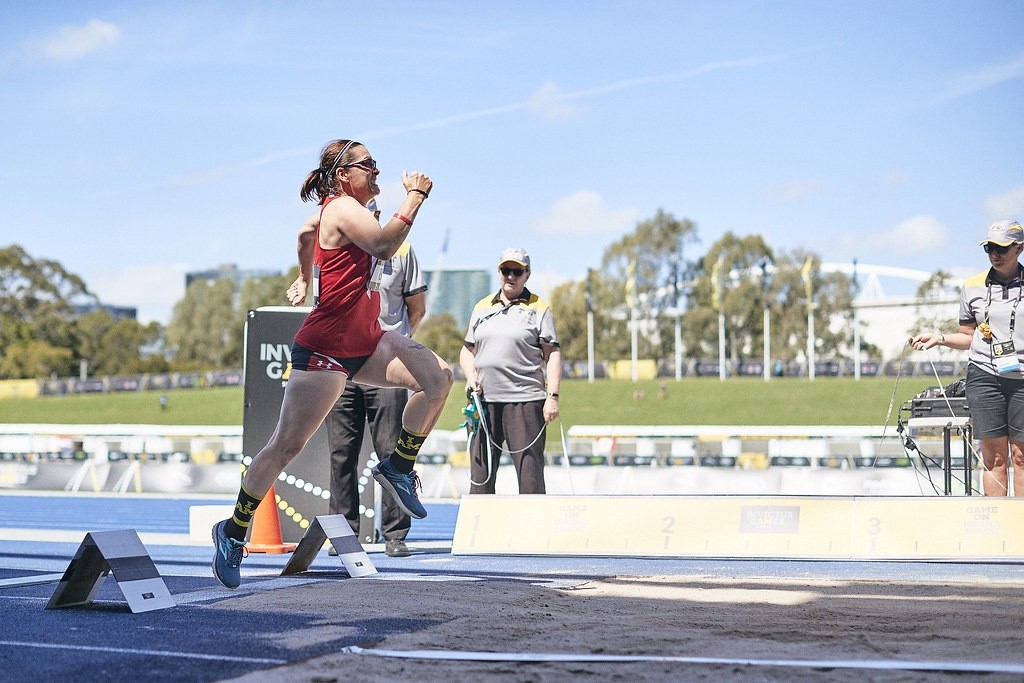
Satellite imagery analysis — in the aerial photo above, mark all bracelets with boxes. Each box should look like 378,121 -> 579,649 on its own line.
547,392 -> 559,397
407,189 -> 428,199
939,333 -> 945,345
393,213 -> 413,227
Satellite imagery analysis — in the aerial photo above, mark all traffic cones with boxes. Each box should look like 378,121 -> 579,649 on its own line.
244,483 -> 295,552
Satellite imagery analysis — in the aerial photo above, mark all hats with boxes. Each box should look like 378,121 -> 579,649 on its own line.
978,218 -> 1024,247
365,196 -> 380,216
496,247 -> 530,269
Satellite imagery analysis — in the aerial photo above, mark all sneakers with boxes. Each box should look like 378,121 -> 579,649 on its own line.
212,518 -> 248,588
372,457 -> 428,520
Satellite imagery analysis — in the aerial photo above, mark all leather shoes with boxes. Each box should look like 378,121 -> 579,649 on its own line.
327,546 -> 337,556
384,539 -> 410,557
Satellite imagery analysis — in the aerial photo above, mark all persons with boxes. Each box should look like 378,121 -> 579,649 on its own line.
908,220 -> 1024,496
459,247 -> 562,494
324,198 -> 429,558
213,137 -> 455,589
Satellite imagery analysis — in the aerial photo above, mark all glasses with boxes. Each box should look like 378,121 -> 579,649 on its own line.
984,244 -> 1015,253
346,159 -> 377,169
501,268 -> 527,276
